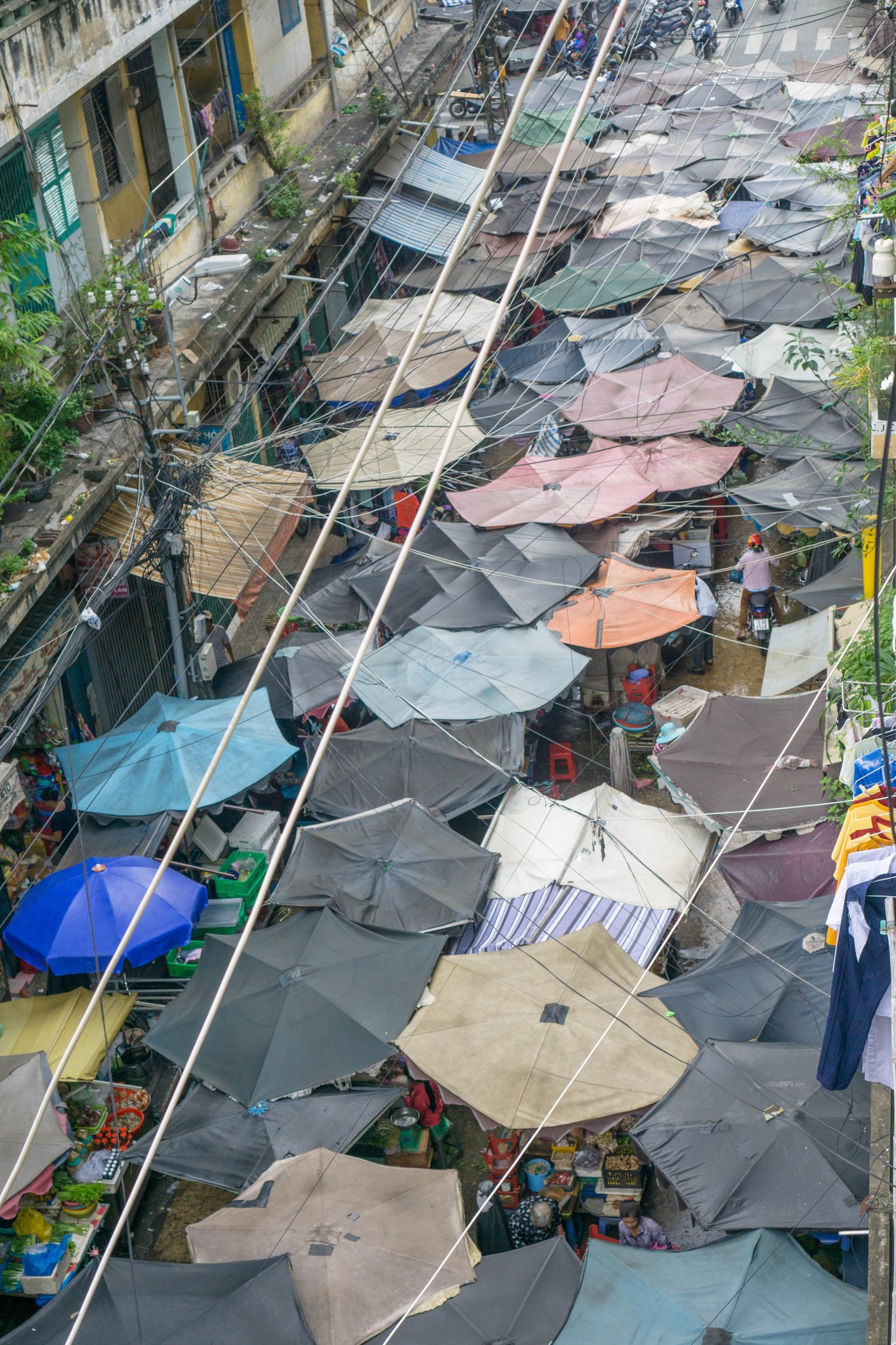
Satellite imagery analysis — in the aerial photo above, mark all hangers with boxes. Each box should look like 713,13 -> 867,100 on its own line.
400,482 -> 413,494
850,694 -> 896,910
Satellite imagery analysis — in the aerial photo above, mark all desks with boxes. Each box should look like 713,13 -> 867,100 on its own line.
385,1129 -> 433,1171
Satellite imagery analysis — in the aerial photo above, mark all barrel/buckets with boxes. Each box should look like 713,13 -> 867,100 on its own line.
525,1158 -> 551,1192
120,1046 -> 154,1085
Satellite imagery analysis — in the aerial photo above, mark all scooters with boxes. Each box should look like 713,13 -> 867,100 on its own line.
723,0 -> 741,28
768,0 -> 784,13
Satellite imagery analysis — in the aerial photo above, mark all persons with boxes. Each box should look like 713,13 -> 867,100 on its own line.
30,787 -> 78,855
346,511 -> 378,547
458,131 -> 469,142
611,0 -> 626,46
618,1197 -> 673,1251
734,533 -> 785,640
530,306 -> 553,338
572,23 -> 599,71
736,0 -> 746,21
687,577 -> 719,675
689,0 -> 713,49
310,708 -> 351,735
203,609 -> 235,668
603,57 -> 621,82
506,1193 -> 559,1249
554,16 -> 571,69
248,770 -> 302,824
401,1062 -> 451,1140
465,125 -> 478,142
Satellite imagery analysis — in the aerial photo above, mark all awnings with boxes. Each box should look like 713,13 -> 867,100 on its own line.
86,434 -> 314,626
347,182 -> 483,265
372,131 -> 494,206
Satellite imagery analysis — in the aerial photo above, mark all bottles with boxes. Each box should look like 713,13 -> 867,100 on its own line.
103,1149 -> 121,1180
199,872 -> 218,900
94,1127 -> 133,1153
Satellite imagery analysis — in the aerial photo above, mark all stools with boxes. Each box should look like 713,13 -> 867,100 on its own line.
480,1132 -> 527,1209
429,1123 -> 464,1169
562,1212 -> 623,1262
620,660 -> 658,711
547,742 -> 578,786
713,512 -> 730,545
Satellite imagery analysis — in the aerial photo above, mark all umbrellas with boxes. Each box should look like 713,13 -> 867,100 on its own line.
0,46 -> 884,1345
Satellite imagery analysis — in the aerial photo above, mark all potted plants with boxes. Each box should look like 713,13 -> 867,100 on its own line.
0,210 -> 175,526
223,83 -> 332,224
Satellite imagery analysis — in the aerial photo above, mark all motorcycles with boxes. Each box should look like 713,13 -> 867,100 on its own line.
690,12 -> 718,61
449,0 -> 696,121
734,556 -> 782,657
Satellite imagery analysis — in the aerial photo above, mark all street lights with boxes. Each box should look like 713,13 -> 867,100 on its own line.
164,252 -> 249,442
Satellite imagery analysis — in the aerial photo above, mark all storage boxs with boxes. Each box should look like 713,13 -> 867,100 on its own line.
543,1131 -> 647,1193
83,1148 -> 131,1195
650,684 -> 710,735
163,809 -> 282,981
18,1242 -> 72,1296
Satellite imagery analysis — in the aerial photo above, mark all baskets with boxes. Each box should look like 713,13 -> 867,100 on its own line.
87,1111 -> 108,1137
349,1120 -> 386,1157
166,941 -> 204,978
0,1209 -> 24,1236
74,1106 -> 107,1133
214,850 -> 268,908
602,1154 -> 645,1189
61,1193 -> 97,1219
104,1108 -> 144,1134
192,899 -> 247,938
